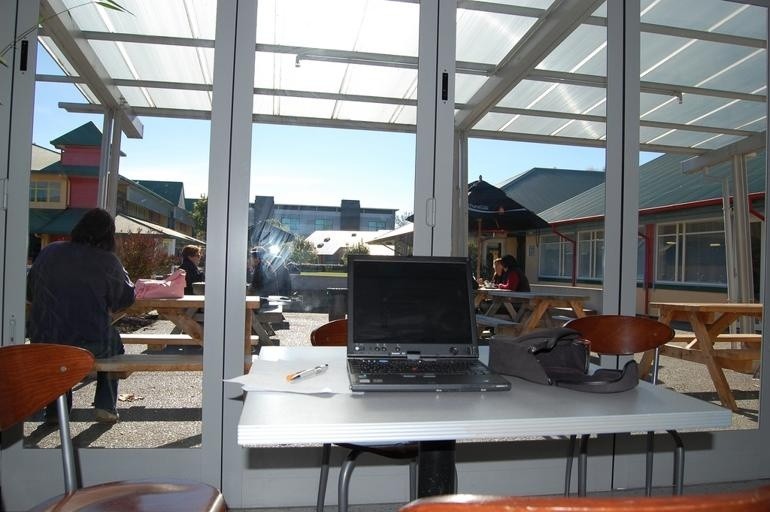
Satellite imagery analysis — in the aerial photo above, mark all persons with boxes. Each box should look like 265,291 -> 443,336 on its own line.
479,255 -> 531,336
176,245 -> 205,314
472,273 -> 479,290
26,207 -> 134,423
479,258 -> 508,288
271,256 -> 292,295
247,245 -> 279,314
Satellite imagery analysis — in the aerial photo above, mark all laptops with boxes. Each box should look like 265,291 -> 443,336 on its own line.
346,255 -> 512,391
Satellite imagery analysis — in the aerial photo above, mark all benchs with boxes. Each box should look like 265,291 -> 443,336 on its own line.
98,333 -> 201,371
476,308 -> 762,376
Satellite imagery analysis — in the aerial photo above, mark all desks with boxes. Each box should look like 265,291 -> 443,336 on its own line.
475,291 -> 591,340
635,301 -> 763,409
236,344 -> 734,512
120,294 -> 261,374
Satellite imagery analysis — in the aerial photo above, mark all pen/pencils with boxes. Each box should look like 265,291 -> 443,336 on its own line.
289,363 -> 328,381
286,369 -> 307,380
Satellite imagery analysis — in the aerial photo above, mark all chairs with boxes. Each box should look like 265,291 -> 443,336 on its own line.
1,344 -> 230,512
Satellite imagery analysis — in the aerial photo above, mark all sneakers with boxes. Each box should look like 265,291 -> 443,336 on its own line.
94,408 -> 120,424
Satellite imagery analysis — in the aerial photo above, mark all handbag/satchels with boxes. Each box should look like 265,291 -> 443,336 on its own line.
487,328 -> 639,394
133,268 -> 187,300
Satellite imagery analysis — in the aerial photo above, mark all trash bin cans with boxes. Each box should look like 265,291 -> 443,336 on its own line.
326,288 -> 348,322
